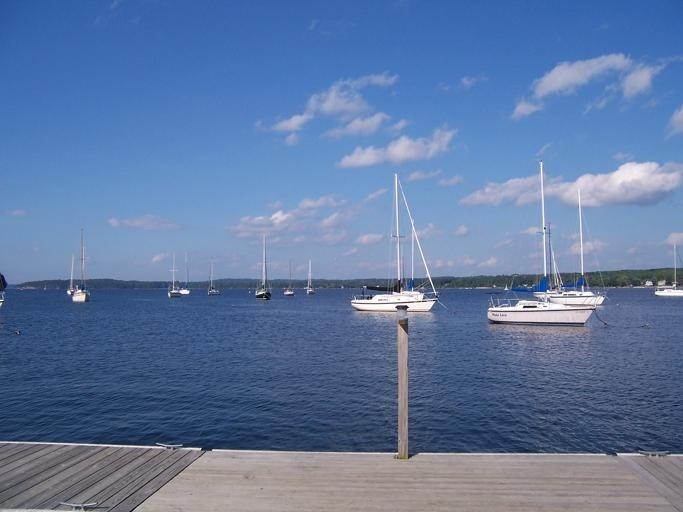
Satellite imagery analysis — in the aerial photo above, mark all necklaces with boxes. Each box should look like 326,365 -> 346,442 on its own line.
487,161 -> 607,324
66,233 -> 90,303
349,173 -> 439,312
303,259 -> 316,295
654,245 -> 682,297
167,251 -> 190,298
283,261 -> 293,296
254,235 -> 271,300
207,264 -> 220,296
